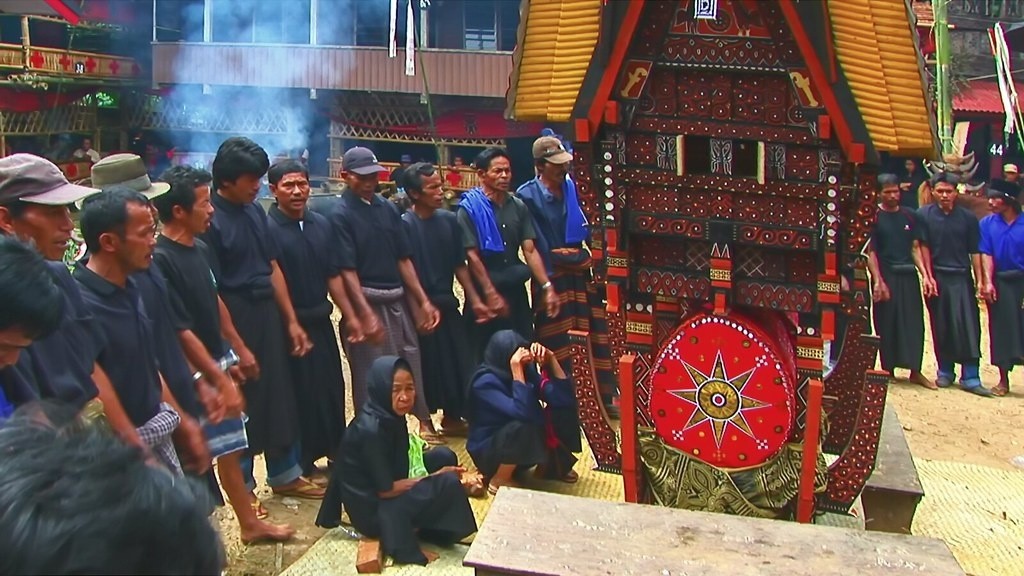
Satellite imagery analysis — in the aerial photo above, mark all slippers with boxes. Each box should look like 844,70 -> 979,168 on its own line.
278,484 -> 326,498
488,487 -> 498,495
420,431 -> 446,444
991,386 -> 1009,396
534,465 -> 579,483
438,423 -> 470,436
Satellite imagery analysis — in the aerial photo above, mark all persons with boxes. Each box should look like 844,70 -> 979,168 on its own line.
1001,165 -> 1024,205
915,170 -> 989,396
865,172 -> 938,390
0,138 -> 614,575
978,176 -> 1024,397
900,157 -> 924,210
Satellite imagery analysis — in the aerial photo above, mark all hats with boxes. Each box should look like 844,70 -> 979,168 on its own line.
342,146 -> 389,176
533,137 -> 573,164
0,152 -> 102,207
1004,164 -> 1018,172
75,152 -> 171,209
401,154 -> 412,162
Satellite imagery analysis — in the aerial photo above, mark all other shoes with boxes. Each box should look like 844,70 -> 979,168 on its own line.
936,375 -> 953,388
970,385 -> 991,396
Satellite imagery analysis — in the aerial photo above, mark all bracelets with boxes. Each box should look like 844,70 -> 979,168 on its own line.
542,280 -> 552,289
191,370 -> 204,383
